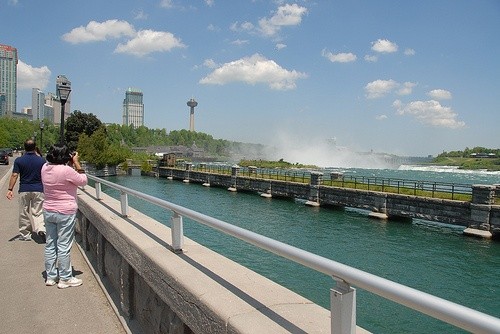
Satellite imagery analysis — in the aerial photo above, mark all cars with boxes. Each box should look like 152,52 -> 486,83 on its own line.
0,150 -> 9,165
4,148 -> 13,156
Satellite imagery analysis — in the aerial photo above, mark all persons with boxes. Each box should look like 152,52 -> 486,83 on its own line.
6,138 -> 47,242
34,145 -> 42,157
38,142 -> 88,289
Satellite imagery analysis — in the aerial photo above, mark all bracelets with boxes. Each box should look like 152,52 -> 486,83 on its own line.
8,189 -> 12,191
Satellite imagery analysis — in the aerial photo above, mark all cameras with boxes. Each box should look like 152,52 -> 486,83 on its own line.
68,153 -> 75,158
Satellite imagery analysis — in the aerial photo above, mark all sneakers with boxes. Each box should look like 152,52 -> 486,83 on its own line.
57,277 -> 83,289
45,278 -> 59,286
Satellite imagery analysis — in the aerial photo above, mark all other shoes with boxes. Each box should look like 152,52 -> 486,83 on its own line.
38,231 -> 46,243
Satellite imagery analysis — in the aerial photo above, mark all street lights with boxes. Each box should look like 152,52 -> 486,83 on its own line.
58,81 -> 72,144
39,120 -> 45,157
